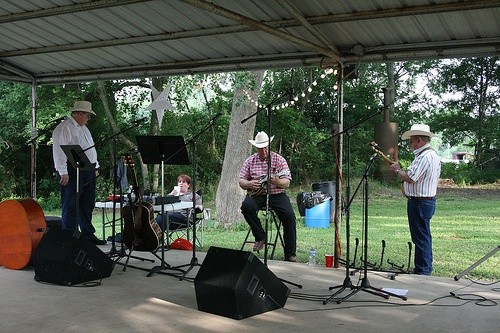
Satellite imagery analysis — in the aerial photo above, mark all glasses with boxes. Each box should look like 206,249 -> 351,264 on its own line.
87,114 -> 91,120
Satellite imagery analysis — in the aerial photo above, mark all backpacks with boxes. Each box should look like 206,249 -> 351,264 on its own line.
170,238 -> 192,251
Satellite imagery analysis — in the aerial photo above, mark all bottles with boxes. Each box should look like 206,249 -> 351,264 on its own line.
309,248 -> 316,266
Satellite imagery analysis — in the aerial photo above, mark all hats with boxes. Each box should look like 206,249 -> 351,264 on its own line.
70,100 -> 96,116
400,123 -> 438,140
247,130 -> 274,149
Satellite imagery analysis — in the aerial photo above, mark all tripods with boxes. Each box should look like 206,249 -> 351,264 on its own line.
83,120 -> 216,281
323,111 -> 409,306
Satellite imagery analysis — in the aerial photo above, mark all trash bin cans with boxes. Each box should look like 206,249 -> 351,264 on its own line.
312,181 -> 336,223
303,191 -> 332,230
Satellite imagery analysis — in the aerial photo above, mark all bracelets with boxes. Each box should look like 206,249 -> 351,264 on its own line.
395,169 -> 401,174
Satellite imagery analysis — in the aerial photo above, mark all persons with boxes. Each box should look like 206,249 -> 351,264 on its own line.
52,101 -> 108,245
154,175 -> 202,233
388,123 -> 442,275
238,130 -> 297,262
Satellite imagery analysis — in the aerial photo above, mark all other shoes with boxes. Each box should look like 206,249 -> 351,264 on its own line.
253,238 -> 266,251
285,256 -> 297,262
80,233 -> 107,245
70,227 -> 80,239
107,235 -> 120,242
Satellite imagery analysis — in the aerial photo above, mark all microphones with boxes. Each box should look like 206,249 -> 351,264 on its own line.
134,117 -> 149,123
209,113 -> 222,123
51,116 -> 68,122
284,88 -> 291,94
375,105 -> 391,111
370,153 -> 378,161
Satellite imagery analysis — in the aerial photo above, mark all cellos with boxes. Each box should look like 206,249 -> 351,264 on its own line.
0,198 -> 47,270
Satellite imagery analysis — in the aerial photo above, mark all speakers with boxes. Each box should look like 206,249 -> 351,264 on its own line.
194,246 -> 291,319
32,215 -> 116,286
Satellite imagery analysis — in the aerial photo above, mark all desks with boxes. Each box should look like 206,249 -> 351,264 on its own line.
95,201 -> 195,251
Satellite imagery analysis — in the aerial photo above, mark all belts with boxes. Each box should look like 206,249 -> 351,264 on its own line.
409,196 -> 433,200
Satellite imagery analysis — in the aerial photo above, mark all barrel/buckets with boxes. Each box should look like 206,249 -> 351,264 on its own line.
311,181 -> 336,223
305,199 -> 329,228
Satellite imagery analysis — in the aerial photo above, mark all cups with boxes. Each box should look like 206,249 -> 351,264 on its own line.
325,255 -> 333,267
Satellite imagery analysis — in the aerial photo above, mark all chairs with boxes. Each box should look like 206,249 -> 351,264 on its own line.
163,189 -> 205,248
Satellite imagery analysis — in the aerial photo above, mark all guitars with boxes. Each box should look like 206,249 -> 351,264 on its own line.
121,152 -> 162,253
245,169 -> 291,197
370,141 -> 410,199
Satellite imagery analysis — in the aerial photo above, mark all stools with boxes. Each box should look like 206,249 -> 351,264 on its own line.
240,207 -> 284,260
